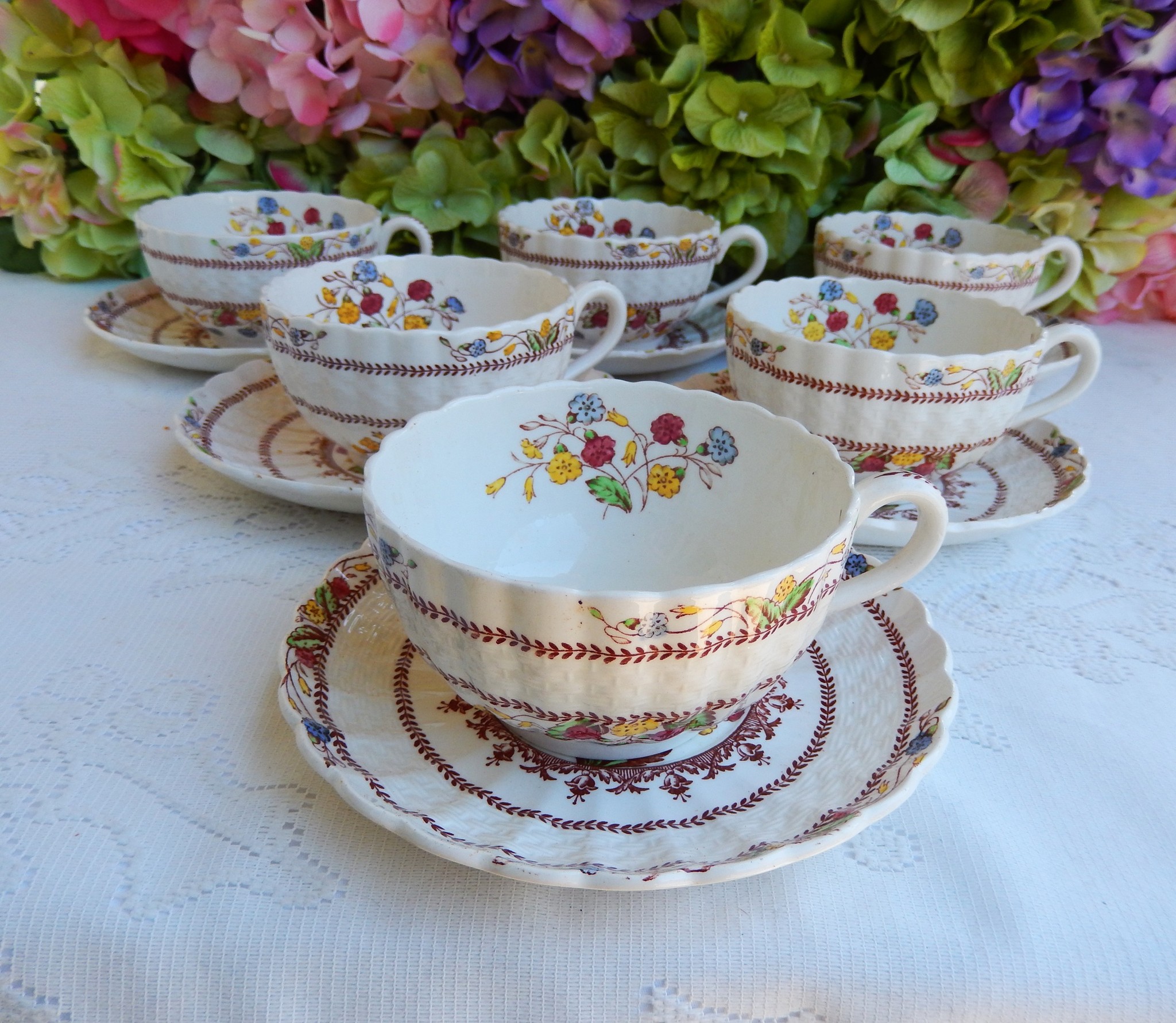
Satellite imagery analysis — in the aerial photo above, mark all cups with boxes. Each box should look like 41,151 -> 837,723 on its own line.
132,189 -> 434,348
814,209 -> 1085,319
497,198 -> 768,346
259,254 -> 626,468
360,380 -> 951,764
726,275 -> 1104,480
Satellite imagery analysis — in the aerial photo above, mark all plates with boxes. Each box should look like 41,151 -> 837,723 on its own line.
171,355 -> 613,512
83,275 -> 274,374
670,366 -> 1089,549
280,535 -> 960,892
1022,310 -> 1080,381
564,305 -> 729,375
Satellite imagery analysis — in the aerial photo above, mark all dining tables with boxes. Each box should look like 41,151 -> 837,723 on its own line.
0,258 -> 1175,1023
